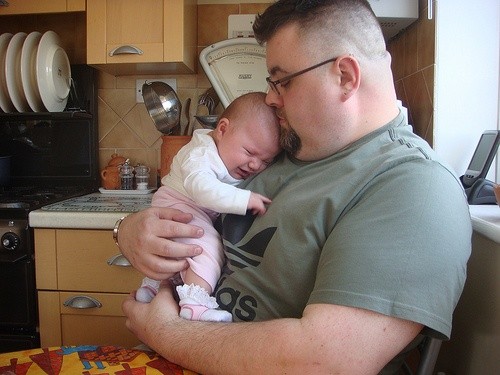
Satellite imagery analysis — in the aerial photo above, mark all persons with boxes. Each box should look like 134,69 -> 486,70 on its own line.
139,0 -> 473,375
140,91 -> 280,324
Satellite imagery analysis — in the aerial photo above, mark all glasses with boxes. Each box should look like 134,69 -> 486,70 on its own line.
265,57 -> 336,96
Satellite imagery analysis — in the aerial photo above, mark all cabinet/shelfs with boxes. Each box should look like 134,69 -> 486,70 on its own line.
35,228 -> 152,347
0,0 -> 196,74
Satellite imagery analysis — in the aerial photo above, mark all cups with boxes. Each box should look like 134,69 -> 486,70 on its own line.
162,136 -> 192,184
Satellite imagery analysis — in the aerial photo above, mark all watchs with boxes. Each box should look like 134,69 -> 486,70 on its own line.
112,215 -> 128,245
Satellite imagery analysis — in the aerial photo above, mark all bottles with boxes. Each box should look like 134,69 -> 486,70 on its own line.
118,161 -> 133,190
135,166 -> 151,189
101,154 -> 127,190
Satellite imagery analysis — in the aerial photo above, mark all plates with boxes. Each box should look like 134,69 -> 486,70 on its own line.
5,32 -> 30,113
0,32 -> 15,114
20,31 -> 47,112
36,30 -> 71,112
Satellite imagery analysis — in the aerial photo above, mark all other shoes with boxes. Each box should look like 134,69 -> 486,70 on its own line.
176,284 -> 234,324
135,276 -> 161,304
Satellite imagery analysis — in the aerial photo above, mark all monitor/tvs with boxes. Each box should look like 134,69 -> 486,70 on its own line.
461,130 -> 500,185
